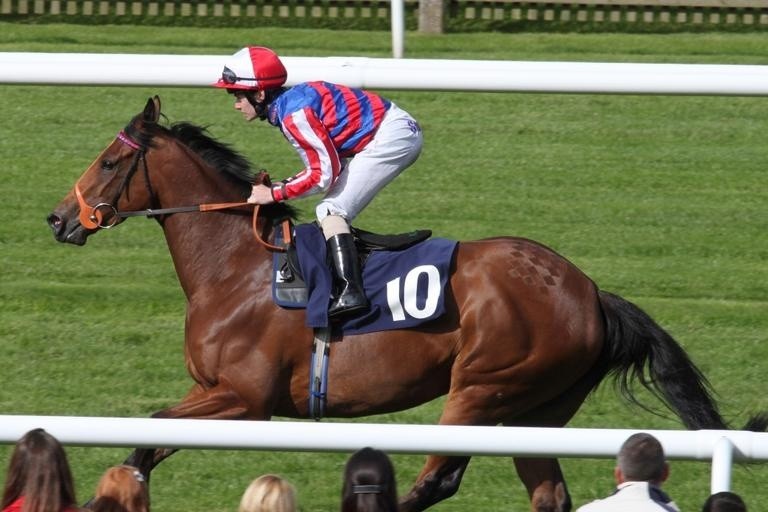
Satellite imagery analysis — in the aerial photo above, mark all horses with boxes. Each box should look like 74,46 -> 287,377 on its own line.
46,92 -> 768,512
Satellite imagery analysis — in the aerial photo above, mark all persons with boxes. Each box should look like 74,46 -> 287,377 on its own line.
0,427 -> 92,512
236,473 -> 298,512
338,448 -> 399,511
78,463 -> 152,512
210,45 -> 423,319
573,433 -> 682,512
702,491 -> 748,512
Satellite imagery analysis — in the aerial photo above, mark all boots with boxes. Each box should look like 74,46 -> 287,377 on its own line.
327,233 -> 371,319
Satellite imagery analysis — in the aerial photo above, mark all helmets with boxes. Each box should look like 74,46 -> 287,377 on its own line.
209,46 -> 287,90
